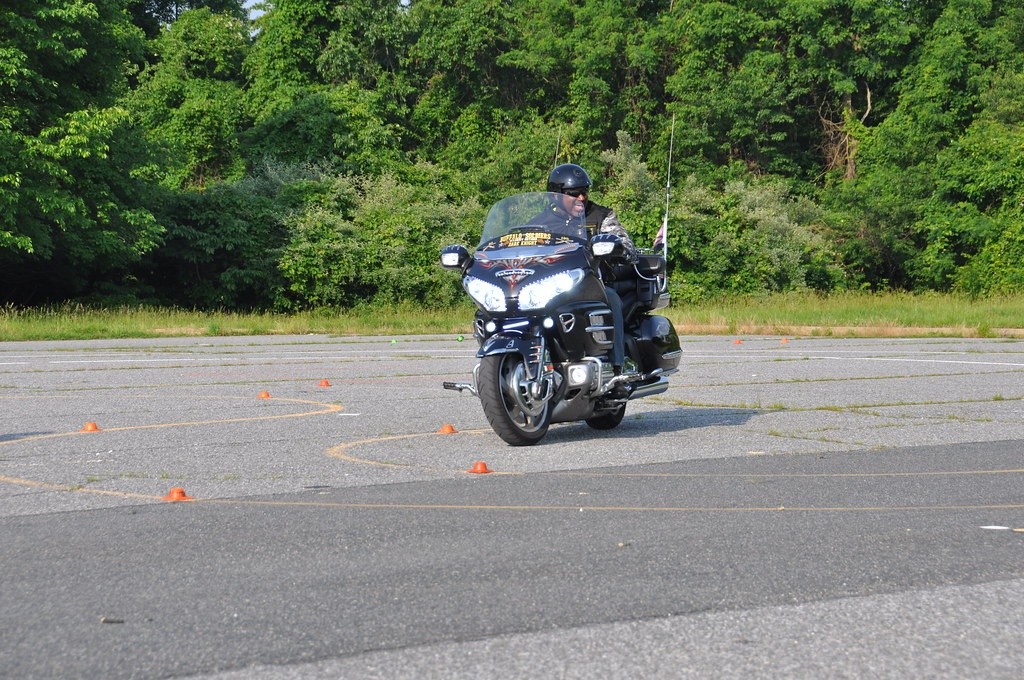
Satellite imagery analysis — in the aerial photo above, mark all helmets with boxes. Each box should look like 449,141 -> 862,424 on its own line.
547,164 -> 591,189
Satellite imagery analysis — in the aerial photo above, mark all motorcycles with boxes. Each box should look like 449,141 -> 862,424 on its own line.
440,191 -> 683,446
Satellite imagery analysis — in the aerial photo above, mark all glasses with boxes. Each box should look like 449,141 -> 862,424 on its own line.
562,190 -> 588,197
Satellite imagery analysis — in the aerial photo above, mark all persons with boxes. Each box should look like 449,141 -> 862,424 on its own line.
523,164 -> 637,402
